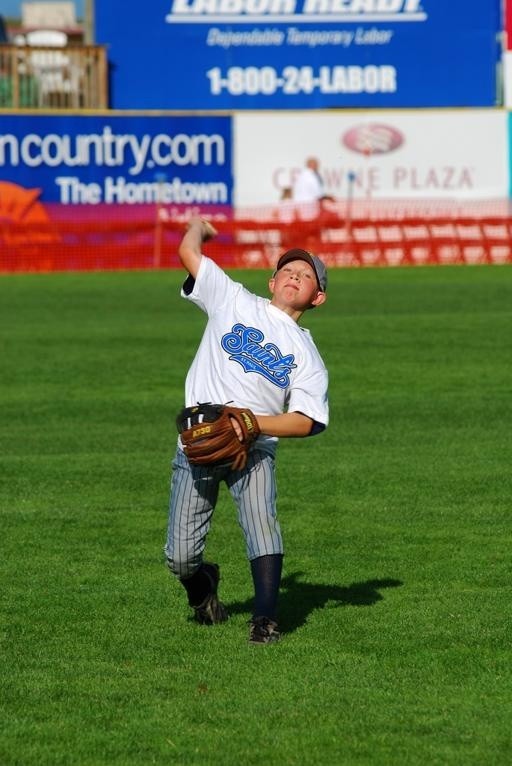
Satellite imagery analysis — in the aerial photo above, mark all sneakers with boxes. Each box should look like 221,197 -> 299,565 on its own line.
186,562 -> 223,624
247,615 -> 278,644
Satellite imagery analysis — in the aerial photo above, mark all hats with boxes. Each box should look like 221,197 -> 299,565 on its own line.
272,248 -> 327,292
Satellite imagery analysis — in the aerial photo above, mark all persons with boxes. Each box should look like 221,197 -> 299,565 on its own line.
168,214 -> 329,645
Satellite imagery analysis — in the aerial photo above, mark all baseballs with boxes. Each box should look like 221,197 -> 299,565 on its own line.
203,219 -> 217,240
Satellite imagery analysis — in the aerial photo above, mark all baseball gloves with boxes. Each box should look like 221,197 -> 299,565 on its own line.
180,404 -> 259,472
